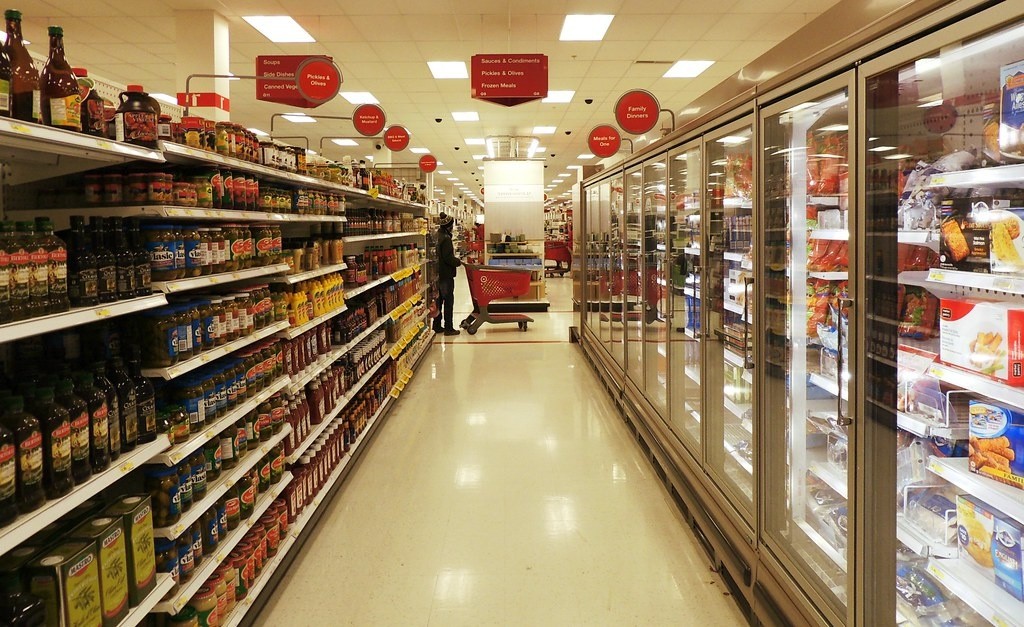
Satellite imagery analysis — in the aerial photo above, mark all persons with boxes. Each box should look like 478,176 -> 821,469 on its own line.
432,211 -> 462,336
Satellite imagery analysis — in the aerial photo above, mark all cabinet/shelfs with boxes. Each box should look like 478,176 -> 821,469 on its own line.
0,0 -> 440,627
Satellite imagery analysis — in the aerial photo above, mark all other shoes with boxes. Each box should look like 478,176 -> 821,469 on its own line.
444,329 -> 460,336
432,327 -> 444,333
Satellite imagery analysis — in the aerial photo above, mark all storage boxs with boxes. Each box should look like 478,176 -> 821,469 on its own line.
981,99 -> 1003,168
490,259 -> 498,265
36,517 -> 82,547
999,58 -> 1024,163
956,494 -> 1024,602
523,259 -> 532,264
0,543 -> 49,596
968,397 -> 1024,490
940,197 -> 1011,273
68,515 -> 129,627
25,537 -> 104,627
67,496 -> 106,517
102,492 -> 157,606
515,259 -> 522,265
506,259 -> 515,265
532,259 -> 542,265
498,259 -> 506,265
988,206 -> 1024,276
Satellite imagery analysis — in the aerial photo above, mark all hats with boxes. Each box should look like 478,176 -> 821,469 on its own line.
440,212 -> 454,229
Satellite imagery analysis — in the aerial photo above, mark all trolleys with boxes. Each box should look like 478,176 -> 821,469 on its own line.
459,260 -> 534,335
